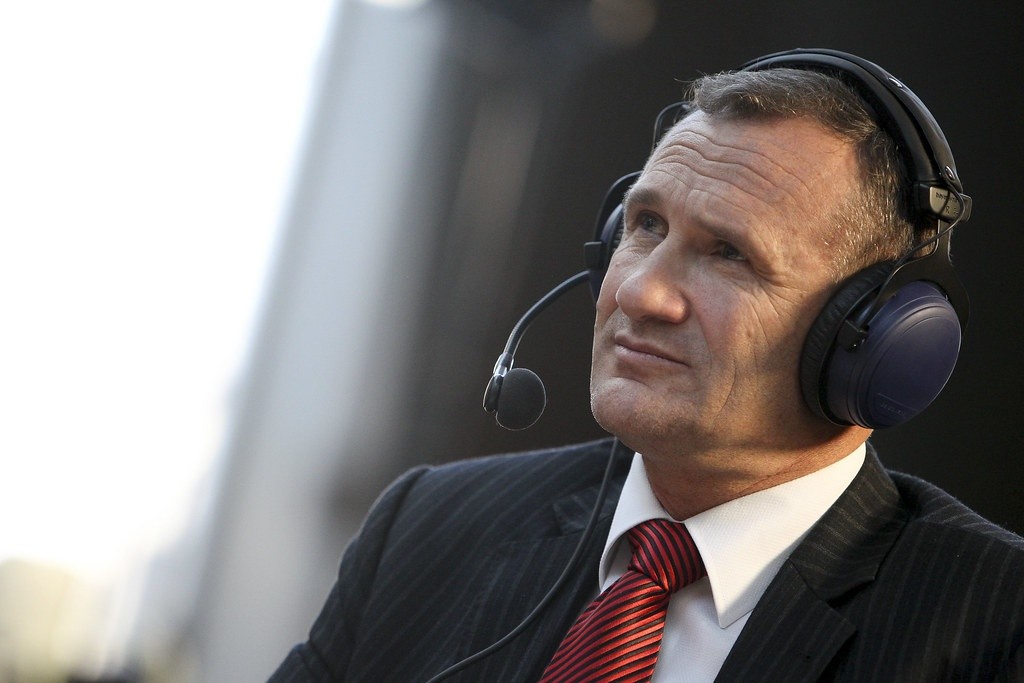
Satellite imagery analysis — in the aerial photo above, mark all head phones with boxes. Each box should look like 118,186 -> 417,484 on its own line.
579,47 -> 977,432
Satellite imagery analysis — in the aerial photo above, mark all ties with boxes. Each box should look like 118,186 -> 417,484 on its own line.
539,518 -> 708,683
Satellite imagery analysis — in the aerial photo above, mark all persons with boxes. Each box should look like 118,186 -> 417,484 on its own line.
266,63 -> 1024,683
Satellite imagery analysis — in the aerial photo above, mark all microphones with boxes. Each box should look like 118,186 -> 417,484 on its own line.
483,270 -> 587,433
